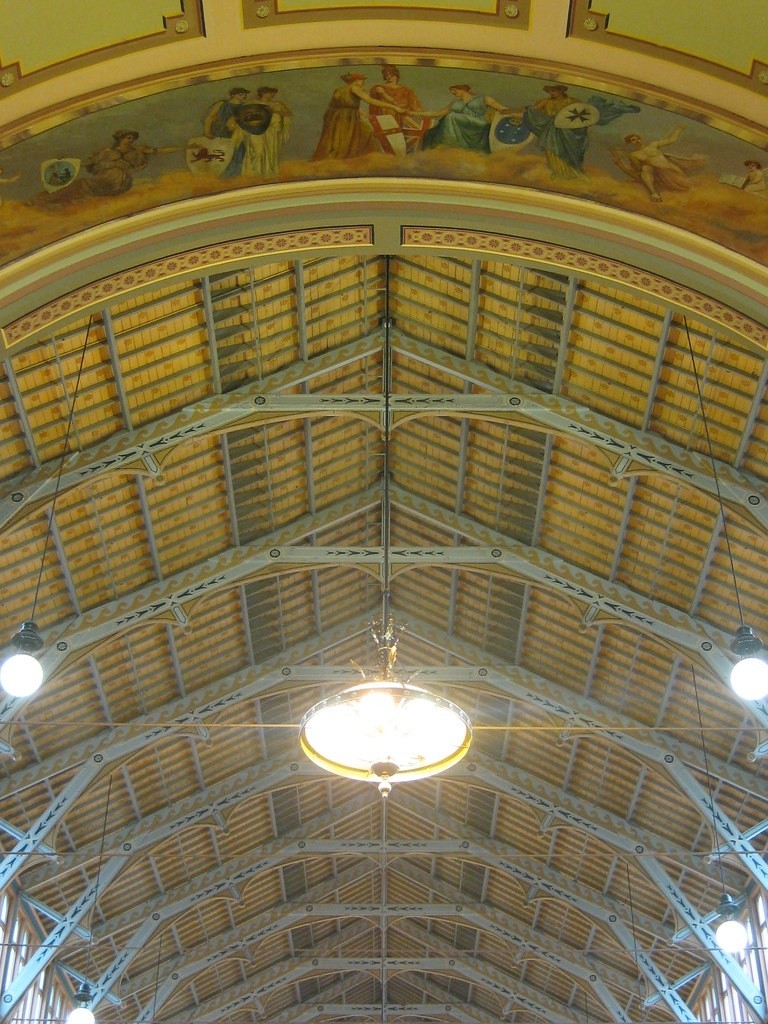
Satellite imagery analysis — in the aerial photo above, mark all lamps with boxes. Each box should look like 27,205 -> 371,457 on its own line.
61,774 -> 114,1024
296,254 -> 474,796
1,317 -> 91,697
682,310 -> 768,700
689,664 -> 750,953
